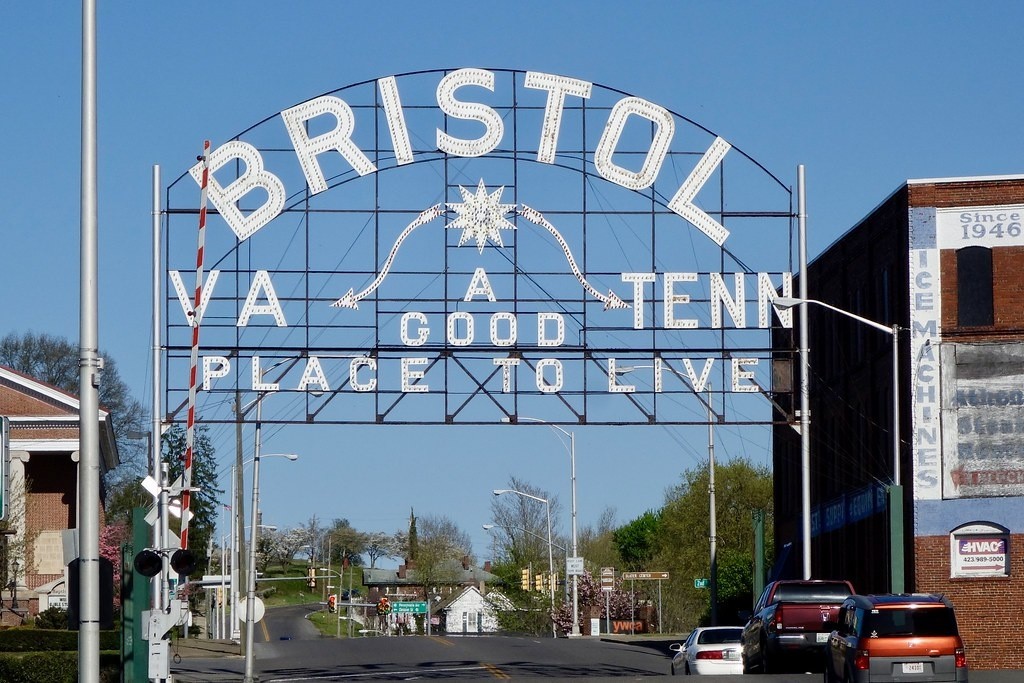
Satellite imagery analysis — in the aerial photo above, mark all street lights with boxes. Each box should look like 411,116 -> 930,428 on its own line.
327,586 -> 352,637
482,524 -> 570,608
772,298 -> 905,597
614,366 -> 718,600
320,568 -> 342,637
230,453 -> 300,641
220,524 -> 278,640
500,416 -> 582,638
493,490 -> 556,638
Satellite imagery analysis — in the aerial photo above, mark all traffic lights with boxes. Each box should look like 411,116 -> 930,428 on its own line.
328,596 -> 337,614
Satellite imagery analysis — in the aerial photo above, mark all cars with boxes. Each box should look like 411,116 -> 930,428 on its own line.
740,580 -> 857,675
669,626 -> 743,675
824,591 -> 969,683
